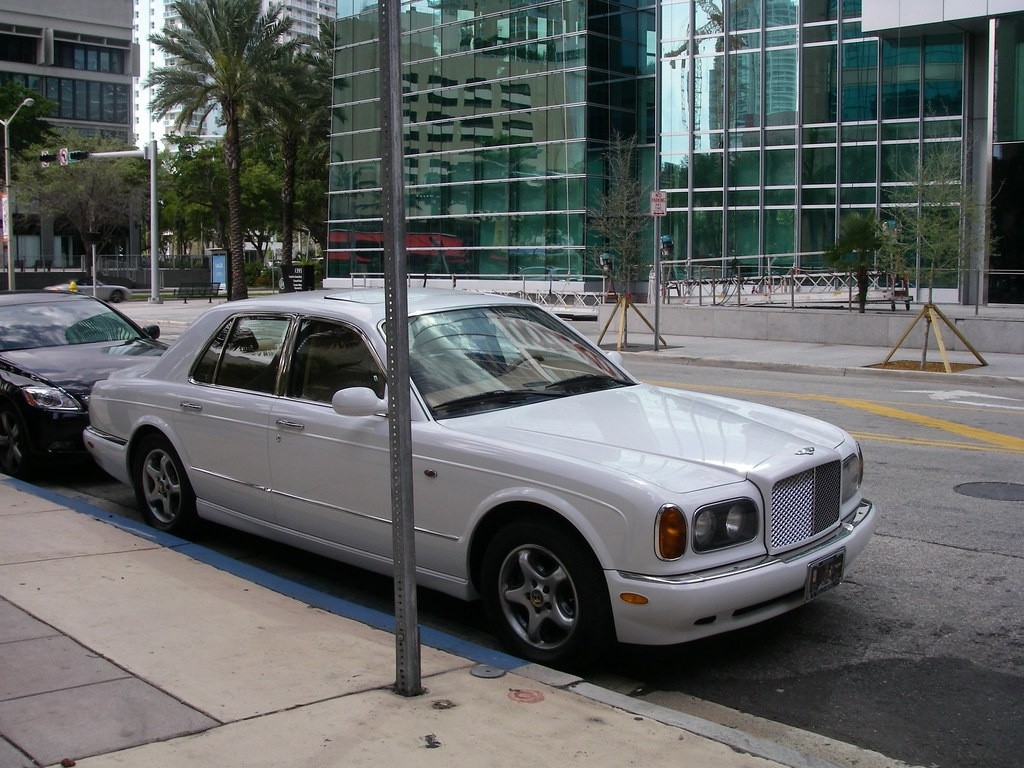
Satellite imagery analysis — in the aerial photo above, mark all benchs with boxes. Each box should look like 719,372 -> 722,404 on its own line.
176,282 -> 221,303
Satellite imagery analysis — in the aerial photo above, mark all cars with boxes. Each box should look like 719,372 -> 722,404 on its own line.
44,276 -> 131,303
518,266 -> 581,280
0,289 -> 179,478
87,286 -> 878,662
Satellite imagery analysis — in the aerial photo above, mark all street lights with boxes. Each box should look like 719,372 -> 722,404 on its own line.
0,98 -> 35,294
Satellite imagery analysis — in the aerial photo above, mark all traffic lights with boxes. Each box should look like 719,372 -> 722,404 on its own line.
69,152 -> 90,162
38,154 -> 57,164
